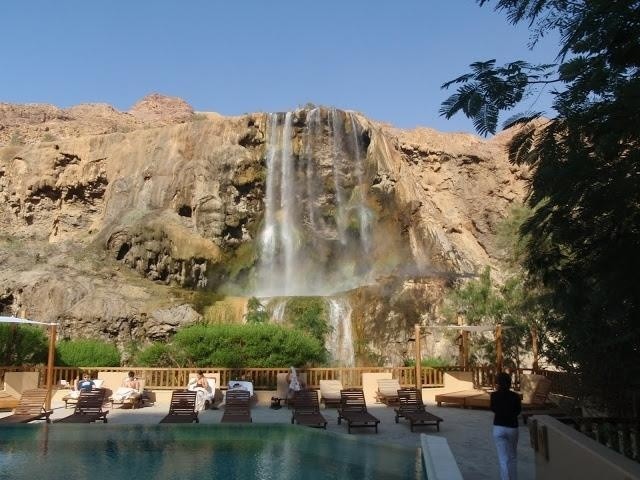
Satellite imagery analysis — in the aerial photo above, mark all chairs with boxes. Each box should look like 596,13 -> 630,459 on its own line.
319,379 -> 347,409
335,387 -> 380,436
394,388 -> 443,433
222,390 -> 253,423
290,390 -> 328,429
272,372 -> 291,409
375,378 -> 411,407
0,372 -> 221,425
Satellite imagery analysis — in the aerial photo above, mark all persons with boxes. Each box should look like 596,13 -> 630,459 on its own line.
74,374 -> 96,398
227,383 -> 243,390
120,371 -> 138,392
187,370 -> 209,392
285,368 -> 304,394
488,373 -> 524,479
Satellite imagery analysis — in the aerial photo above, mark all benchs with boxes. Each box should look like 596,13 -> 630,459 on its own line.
464,392 -> 492,412
435,389 -> 485,408
